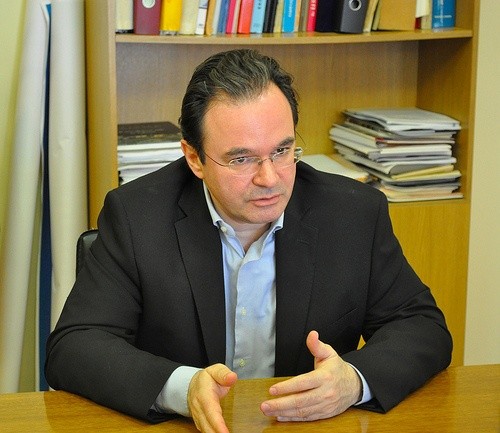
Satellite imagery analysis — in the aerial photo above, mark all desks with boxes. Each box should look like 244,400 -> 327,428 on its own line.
0,363 -> 500,433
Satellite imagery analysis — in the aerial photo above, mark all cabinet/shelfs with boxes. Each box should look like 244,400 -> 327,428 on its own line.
85,0 -> 480,366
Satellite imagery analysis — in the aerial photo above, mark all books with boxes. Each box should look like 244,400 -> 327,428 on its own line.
114,0 -> 455,37
297,109 -> 462,202
117,121 -> 184,186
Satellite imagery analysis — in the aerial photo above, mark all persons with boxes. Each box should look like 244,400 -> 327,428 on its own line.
43,49 -> 454,433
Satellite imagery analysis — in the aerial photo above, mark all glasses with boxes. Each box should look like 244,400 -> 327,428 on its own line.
202,131 -> 306,177
